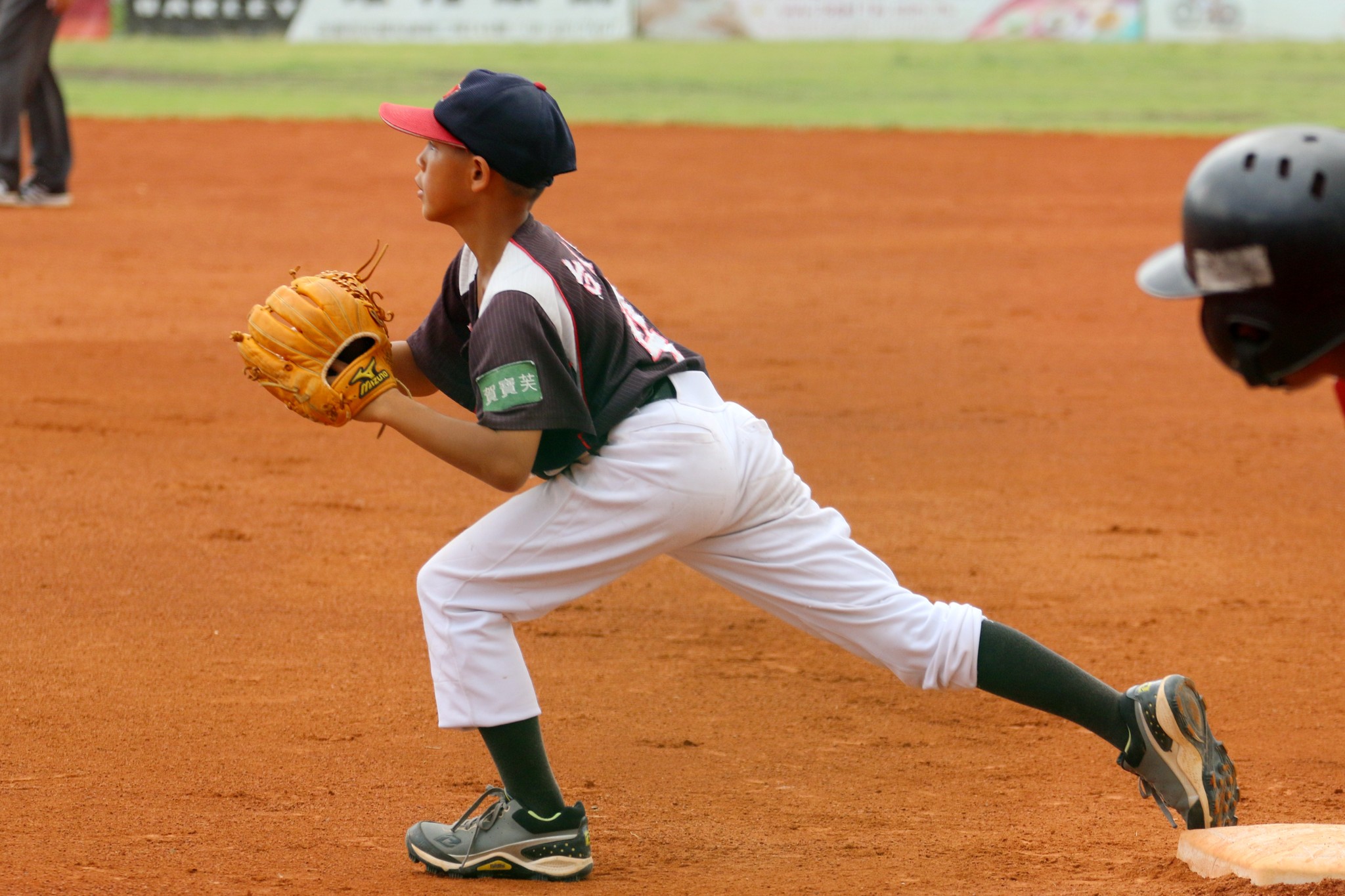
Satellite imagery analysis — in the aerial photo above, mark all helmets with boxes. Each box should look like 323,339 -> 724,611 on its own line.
1133,121 -> 1344,392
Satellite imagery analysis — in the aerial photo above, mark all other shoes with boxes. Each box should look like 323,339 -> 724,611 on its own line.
18,179 -> 71,209
0,179 -> 22,206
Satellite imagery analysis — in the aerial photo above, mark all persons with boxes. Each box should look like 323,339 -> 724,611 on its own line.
228,70 -> 1242,882
0,0 -> 81,207
1135,124 -> 1344,419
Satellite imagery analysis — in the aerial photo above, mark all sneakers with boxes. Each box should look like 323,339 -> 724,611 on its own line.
1117,673 -> 1241,830
403,785 -> 592,882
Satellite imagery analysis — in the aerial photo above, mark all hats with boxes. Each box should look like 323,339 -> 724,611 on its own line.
379,68 -> 577,188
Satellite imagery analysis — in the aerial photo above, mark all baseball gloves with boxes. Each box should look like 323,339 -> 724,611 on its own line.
232,267 -> 403,426
